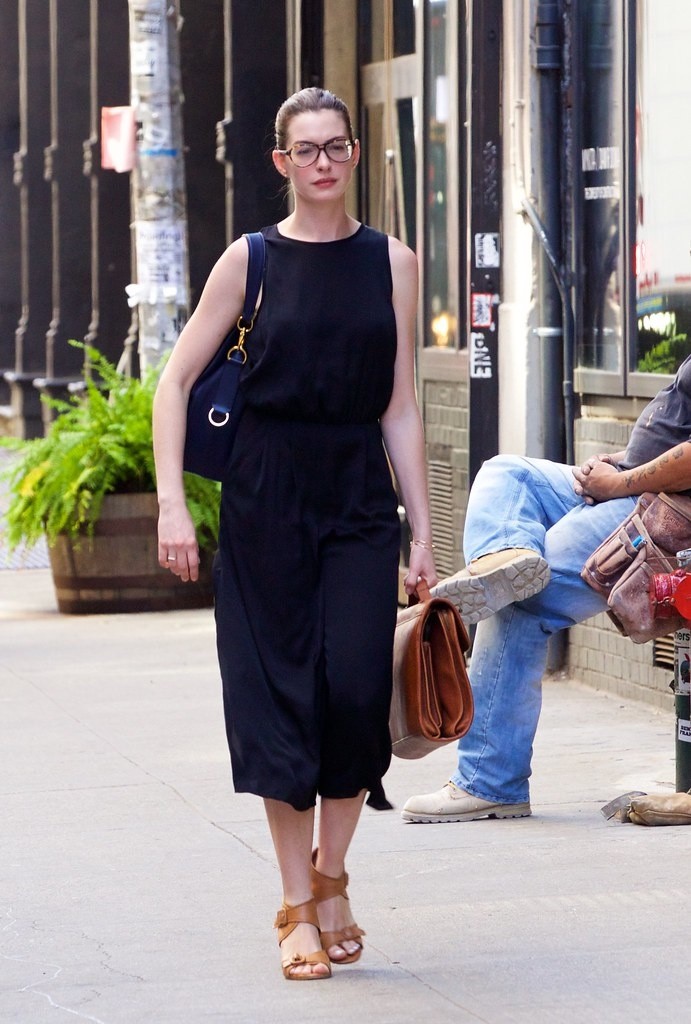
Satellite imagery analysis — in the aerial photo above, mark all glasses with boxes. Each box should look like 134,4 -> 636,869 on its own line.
283,136 -> 355,169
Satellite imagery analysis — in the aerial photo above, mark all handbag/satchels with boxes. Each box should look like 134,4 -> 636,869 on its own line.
181,232 -> 269,486
598,790 -> 691,826
390,573 -> 473,762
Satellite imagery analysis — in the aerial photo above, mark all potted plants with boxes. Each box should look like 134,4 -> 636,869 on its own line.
0,338 -> 222,614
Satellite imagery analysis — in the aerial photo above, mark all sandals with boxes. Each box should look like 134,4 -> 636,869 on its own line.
311,850 -> 366,966
273,895 -> 332,982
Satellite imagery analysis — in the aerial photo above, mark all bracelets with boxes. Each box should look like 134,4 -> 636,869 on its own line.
410,538 -> 436,552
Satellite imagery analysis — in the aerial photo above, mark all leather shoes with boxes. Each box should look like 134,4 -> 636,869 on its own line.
428,550 -> 552,625
402,783 -> 531,825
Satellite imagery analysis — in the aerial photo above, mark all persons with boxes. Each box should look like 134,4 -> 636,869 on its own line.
401,352 -> 691,822
152,88 -> 437,981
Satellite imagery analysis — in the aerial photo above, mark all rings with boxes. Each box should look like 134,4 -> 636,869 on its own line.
168,556 -> 176,561
